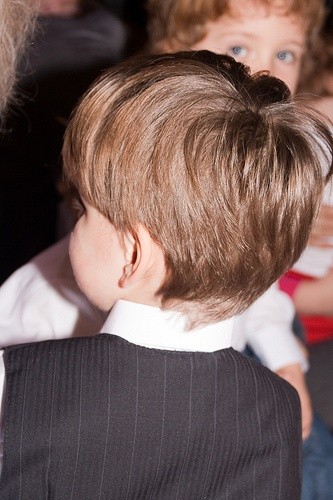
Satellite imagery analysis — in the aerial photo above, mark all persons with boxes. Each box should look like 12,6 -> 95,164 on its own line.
0,0 -> 333,500
